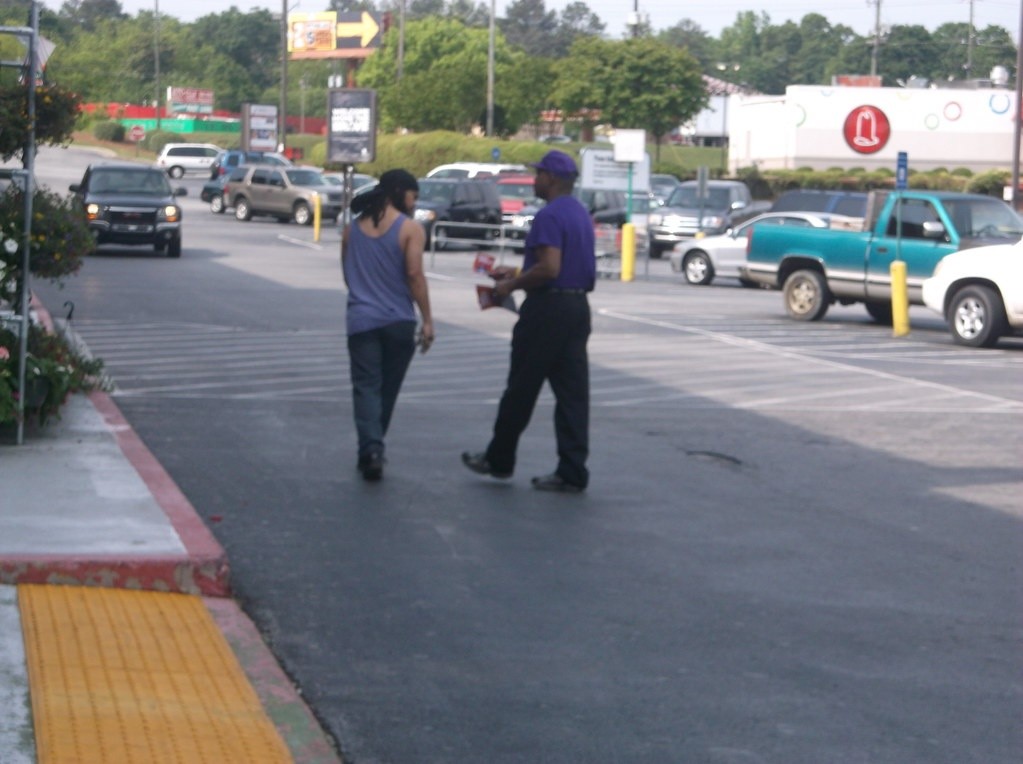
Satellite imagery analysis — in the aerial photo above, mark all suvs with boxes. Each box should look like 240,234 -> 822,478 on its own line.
646,180 -> 776,260
412,176 -> 503,251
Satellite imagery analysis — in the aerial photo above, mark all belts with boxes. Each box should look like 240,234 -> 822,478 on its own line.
544,287 -> 586,295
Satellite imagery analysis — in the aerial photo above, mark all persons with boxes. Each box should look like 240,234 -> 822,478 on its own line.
462,151 -> 596,491
341,169 -> 434,479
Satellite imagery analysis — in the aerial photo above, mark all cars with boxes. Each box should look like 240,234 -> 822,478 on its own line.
203,149 -> 895,234
921,233 -> 1023,349
155,142 -> 227,181
222,164 -> 344,226
669,210 -> 865,291
68,159 -> 188,259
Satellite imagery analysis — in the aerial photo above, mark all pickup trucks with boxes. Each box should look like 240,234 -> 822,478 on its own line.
745,187 -> 1023,328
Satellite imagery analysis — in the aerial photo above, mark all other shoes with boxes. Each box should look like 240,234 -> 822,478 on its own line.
462,452 -> 513,478
532,473 -> 580,492
357,452 -> 382,479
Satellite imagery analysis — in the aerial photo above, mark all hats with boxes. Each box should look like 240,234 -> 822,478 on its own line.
529,149 -> 578,181
